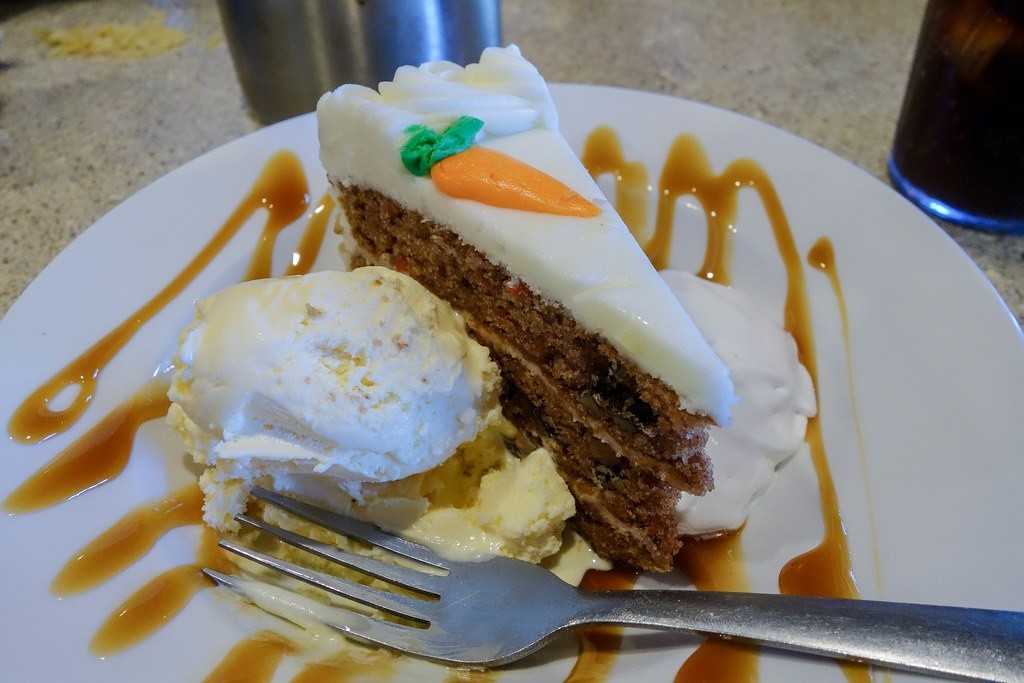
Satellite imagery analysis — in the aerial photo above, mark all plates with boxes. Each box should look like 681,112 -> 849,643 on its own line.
0,83 -> 1024,683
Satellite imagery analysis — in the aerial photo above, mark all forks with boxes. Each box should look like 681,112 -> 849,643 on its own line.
202,488 -> 1024,683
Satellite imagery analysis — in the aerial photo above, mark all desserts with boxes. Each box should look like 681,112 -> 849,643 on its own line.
170,47 -> 813,633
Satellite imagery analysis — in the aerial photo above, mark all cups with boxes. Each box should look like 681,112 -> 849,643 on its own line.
890,0 -> 1024,230
220,0 -> 503,128
